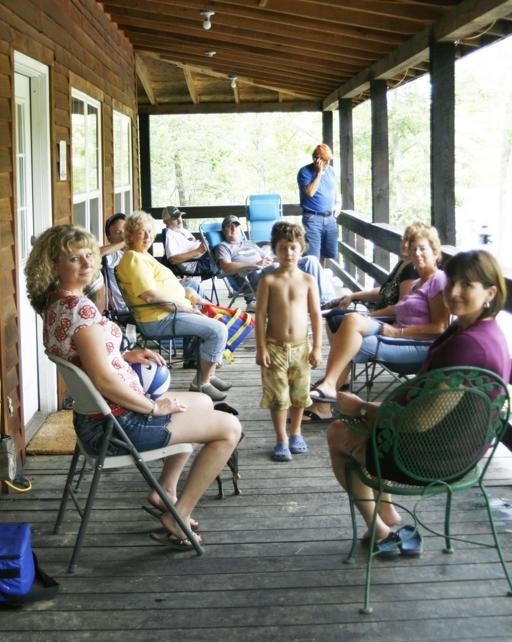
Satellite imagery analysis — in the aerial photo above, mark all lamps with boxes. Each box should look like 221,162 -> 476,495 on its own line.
198,10 -> 214,30
230,71 -> 237,89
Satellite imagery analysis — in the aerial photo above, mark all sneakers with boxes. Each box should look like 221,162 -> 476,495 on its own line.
189,384 -> 226,401
210,377 -> 232,392
183,359 -> 197,369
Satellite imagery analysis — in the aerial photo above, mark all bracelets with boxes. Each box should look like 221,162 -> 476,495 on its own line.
400,327 -> 404,338
144,400 -> 158,421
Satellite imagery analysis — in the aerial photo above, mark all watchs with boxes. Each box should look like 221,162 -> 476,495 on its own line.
359,402 -> 369,417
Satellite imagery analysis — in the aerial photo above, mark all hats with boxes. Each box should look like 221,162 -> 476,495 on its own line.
222,215 -> 240,229
162,206 -> 187,221
106,213 -> 126,229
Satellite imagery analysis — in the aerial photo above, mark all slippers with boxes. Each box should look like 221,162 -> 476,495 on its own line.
309,388 -> 338,402
141,504 -> 198,530
395,525 -> 424,555
151,528 -> 204,551
377,532 -> 400,557
290,435 -> 307,453
274,442 -> 292,462
287,410 -> 332,424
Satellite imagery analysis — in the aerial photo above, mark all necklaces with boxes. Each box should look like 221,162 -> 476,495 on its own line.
57,282 -> 85,295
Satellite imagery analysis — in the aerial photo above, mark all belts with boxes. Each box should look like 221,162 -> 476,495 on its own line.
303,208 -> 335,217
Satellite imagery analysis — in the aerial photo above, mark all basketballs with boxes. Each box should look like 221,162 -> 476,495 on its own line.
128,356 -> 174,400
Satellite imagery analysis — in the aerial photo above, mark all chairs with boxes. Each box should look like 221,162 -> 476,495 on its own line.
58,354 -> 207,576
160,228 -> 221,308
339,366 -> 512,616
198,218 -> 261,309
113,263 -> 204,393
245,194 -> 283,246
342,333 -> 434,399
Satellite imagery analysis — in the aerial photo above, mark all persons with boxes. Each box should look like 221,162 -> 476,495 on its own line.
24,224 -> 242,545
216,214 -> 335,306
98,213 -> 206,369
310,221 -> 439,390
162,205 -> 219,275
117,210 -> 232,401
326,249 -> 511,541
255,221 -> 323,460
287,228 -> 449,424
297,143 -> 342,263
